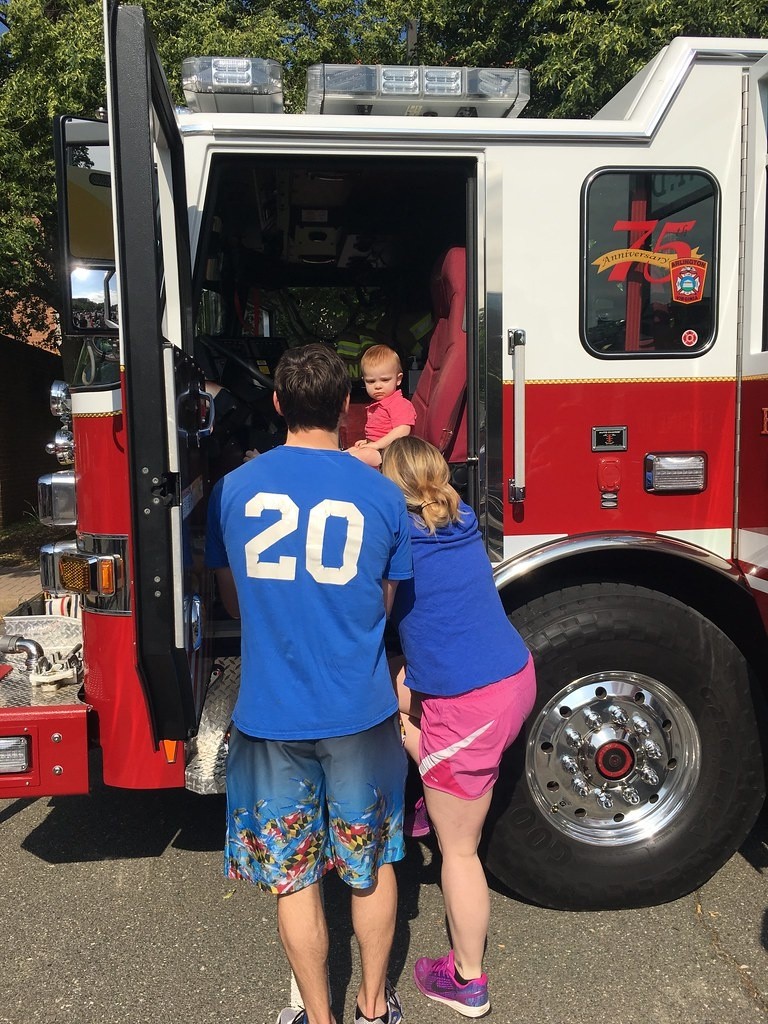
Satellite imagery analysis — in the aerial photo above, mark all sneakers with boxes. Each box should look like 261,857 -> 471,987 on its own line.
413,950 -> 491,1018
276,1005 -> 309,1024
353,976 -> 404,1024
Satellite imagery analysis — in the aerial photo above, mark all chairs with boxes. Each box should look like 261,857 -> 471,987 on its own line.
338,248 -> 466,495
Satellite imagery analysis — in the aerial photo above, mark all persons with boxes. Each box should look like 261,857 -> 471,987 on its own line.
208,345 -> 535,1024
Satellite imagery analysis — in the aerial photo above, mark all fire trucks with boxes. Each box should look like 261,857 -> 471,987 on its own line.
0,34 -> 767,913
